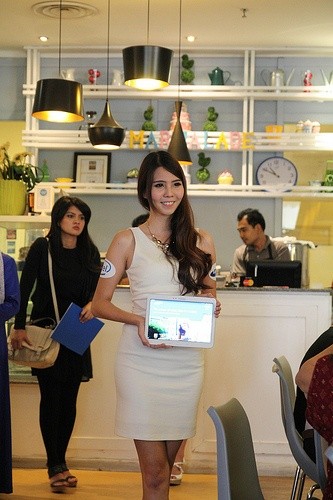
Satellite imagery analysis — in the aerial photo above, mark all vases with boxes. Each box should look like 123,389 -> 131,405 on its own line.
0,179 -> 28,215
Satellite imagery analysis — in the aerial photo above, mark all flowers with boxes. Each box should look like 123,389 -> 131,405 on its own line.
0,141 -> 45,192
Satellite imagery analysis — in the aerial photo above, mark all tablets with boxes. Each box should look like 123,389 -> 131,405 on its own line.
146,294 -> 217,350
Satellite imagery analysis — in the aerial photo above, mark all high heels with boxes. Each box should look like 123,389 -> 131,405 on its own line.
63,468 -> 77,487
169,458 -> 185,485
49,474 -> 69,493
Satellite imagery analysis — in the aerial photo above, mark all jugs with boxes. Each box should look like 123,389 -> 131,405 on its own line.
111,70 -> 124,85
261,67 -> 296,92
61,68 -> 75,81
208,68 -> 231,85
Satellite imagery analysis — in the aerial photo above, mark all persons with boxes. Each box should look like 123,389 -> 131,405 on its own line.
293,327 -> 333,499
119,214 -> 149,284
0,253 -> 21,494
12,196 -> 102,492
91,151 -> 222,500
233,208 -> 291,274
170,439 -> 188,485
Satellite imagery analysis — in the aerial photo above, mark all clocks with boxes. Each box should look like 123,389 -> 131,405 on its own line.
256,156 -> 299,192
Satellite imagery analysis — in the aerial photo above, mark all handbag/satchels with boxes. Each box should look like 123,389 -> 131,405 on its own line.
6,323 -> 60,369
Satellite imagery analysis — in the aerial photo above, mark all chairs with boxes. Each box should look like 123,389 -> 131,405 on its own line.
208,398 -> 265,500
271,356 -> 333,500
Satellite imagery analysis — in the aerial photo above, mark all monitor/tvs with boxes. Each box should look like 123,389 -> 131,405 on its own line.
245,261 -> 302,288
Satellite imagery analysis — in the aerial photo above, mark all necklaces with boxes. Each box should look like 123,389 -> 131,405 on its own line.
147,220 -> 169,247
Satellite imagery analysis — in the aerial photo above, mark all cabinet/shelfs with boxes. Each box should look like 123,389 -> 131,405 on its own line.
21,45 -> 333,199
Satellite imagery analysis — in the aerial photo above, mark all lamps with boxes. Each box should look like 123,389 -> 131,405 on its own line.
166,0 -> 191,165
87,0 -> 125,148
120,0 -> 172,93
30,0 -> 84,125
